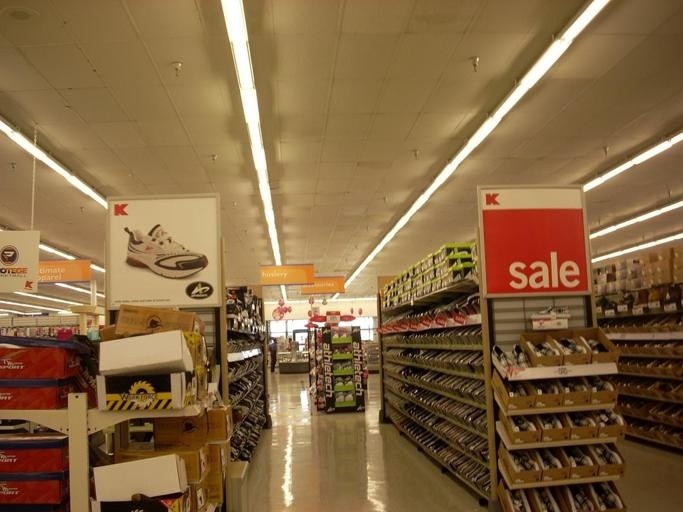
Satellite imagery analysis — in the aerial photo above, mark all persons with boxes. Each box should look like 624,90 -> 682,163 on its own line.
269,338 -> 277,372
286,338 -> 292,352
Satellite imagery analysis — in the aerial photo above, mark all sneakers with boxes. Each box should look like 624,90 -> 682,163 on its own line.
493,337 -> 623,512
124,225 -> 208,279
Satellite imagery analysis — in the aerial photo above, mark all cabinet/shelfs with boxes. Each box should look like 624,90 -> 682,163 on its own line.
306,321 -> 369,415
0,285 -> 271,512
277,350 -> 309,375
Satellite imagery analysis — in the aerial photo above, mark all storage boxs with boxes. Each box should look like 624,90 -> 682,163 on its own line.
376,240 -> 626,512
626,240 -> 683,451
